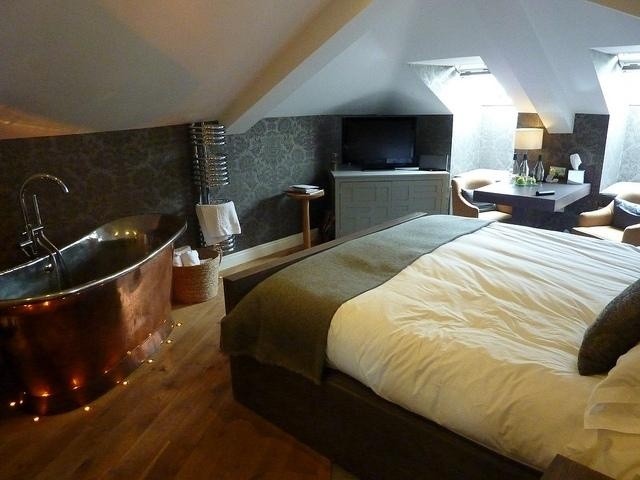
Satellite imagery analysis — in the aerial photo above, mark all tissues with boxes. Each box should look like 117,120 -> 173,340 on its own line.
566,153 -> 586,186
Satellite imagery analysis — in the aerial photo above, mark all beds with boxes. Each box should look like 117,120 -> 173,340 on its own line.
224,211 -> 640,479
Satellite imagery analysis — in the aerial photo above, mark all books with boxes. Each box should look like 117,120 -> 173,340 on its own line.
287,185 -> 319,194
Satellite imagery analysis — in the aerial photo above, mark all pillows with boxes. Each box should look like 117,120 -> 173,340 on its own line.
462,188 -> 498,212
579,278 -> 640,436
612,197 -> 640,228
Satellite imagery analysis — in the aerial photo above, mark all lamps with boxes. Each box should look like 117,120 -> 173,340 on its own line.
512,128 -> 544,150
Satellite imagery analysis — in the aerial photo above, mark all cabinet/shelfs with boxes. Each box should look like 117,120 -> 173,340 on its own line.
329,170 -> 451,240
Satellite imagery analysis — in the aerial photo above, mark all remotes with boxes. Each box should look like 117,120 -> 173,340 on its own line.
536,191 -> 555,195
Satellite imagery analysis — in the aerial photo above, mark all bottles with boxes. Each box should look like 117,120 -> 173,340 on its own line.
519,153 -> 530,177
533,155 -> 545,181
508,153 -> 520,184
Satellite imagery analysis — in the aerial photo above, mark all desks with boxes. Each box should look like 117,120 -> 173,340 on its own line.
474,179 -> 591,212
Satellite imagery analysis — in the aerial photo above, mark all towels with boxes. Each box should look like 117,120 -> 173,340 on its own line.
196,201 -> 242,246
173,245 -> 212,267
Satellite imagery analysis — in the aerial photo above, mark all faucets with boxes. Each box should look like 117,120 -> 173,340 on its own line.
17,173 -> 69,258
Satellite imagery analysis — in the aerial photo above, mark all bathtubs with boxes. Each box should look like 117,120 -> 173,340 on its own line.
0,211 -> 188,415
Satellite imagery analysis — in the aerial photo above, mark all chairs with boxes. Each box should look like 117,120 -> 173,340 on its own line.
572,190 -> 640,248
451,168 -> 512,223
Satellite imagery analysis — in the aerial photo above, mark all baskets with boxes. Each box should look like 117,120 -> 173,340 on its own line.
172,248 -> 221,304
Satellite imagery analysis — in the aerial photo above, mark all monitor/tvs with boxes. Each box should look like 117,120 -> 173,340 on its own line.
341,114 -> 419,171
